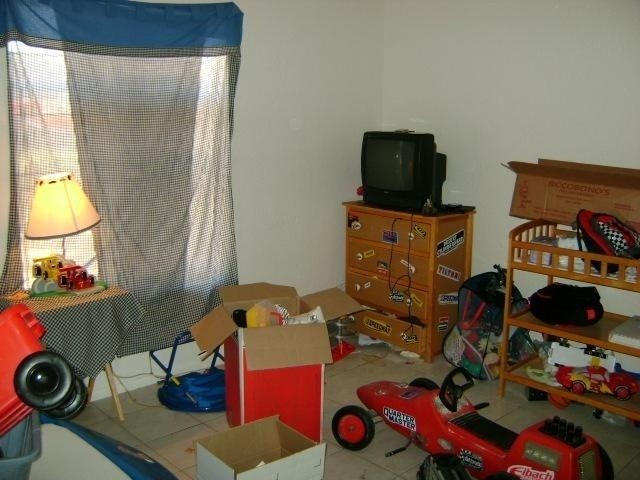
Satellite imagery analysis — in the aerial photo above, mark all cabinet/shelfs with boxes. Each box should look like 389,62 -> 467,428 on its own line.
341,199 -> 478,367
496,217 -> 640,426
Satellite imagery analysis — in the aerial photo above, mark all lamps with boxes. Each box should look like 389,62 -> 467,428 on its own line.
24,171 -> 107,300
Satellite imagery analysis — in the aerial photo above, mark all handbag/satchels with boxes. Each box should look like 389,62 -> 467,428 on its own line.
529,282 -> 604,326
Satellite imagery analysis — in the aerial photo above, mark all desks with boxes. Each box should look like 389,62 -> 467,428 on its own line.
0,286 -> 148,425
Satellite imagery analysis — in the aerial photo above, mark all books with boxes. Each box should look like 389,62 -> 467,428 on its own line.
608,314 -> 640,350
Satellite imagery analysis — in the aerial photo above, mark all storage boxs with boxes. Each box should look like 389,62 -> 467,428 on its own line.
191,413 -> 329,480
501,158 -> 640,234
187,280 -> 368,444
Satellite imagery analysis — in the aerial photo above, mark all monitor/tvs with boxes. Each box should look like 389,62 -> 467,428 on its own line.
359,131 -> 449,212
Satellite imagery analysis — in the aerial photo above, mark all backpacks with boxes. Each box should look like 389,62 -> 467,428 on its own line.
577,208 -> 640,273
